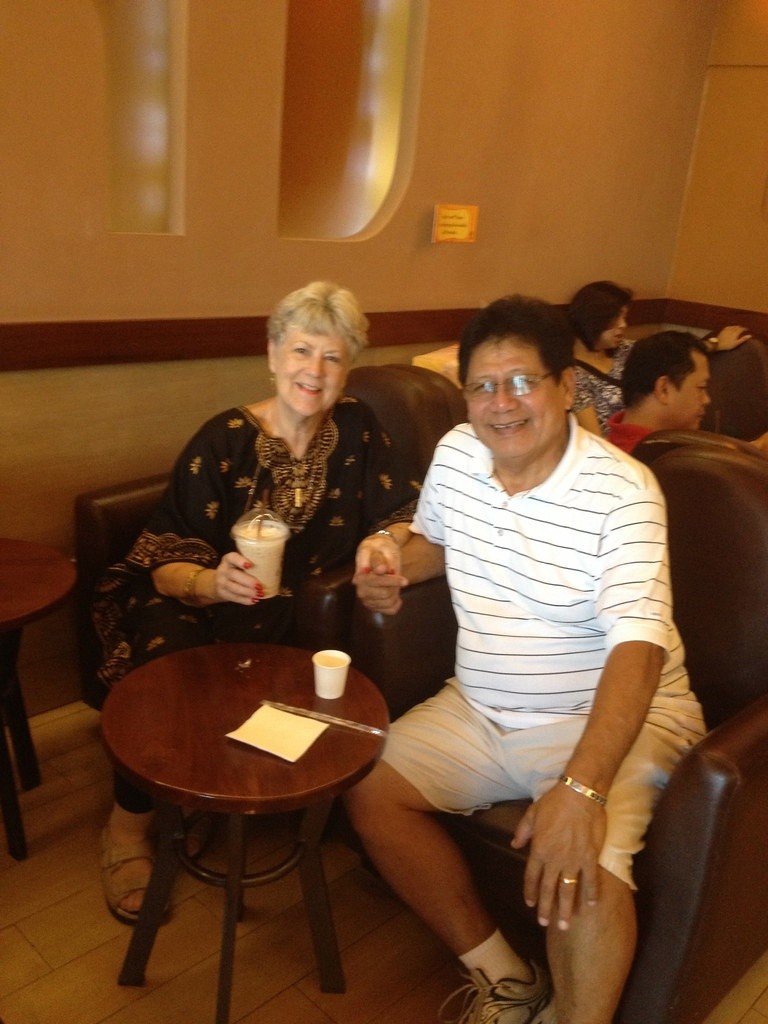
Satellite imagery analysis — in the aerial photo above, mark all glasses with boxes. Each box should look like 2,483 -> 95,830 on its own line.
463,371 -> 556,402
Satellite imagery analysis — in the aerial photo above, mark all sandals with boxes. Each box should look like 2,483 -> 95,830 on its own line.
99,824 -> 169,925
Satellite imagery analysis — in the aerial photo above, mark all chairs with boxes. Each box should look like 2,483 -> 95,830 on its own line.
80,327 -> 768,1024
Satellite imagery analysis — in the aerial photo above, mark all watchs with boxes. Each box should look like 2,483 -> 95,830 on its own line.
709,337 -> 720,351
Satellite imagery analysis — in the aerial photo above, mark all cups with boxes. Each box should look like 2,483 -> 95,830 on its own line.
312,649 -> 352,702
232,508 -> 292,599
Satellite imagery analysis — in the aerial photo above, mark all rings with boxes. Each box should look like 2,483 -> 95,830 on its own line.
558,875 -> 580,885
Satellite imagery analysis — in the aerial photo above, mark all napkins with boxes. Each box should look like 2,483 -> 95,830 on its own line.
225,704 -> 330,763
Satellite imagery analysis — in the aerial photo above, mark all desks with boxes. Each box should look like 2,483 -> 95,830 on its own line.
100,641 -> 389,1024
0,538 -> 78,861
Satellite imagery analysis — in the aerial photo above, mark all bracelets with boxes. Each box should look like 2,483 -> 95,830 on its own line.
558,772 -> 610,809
182,568 -> 208,601
374,530 -> 399,543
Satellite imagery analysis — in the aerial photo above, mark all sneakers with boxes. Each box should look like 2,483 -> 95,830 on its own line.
437,953 -> 553,1024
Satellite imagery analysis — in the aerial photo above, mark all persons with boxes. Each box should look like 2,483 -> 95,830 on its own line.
352,294 -> 709,1023
76,279 -> 422,925
570,279 -> 767,454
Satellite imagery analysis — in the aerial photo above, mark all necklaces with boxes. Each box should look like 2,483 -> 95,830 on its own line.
293,488 -> 304,508
